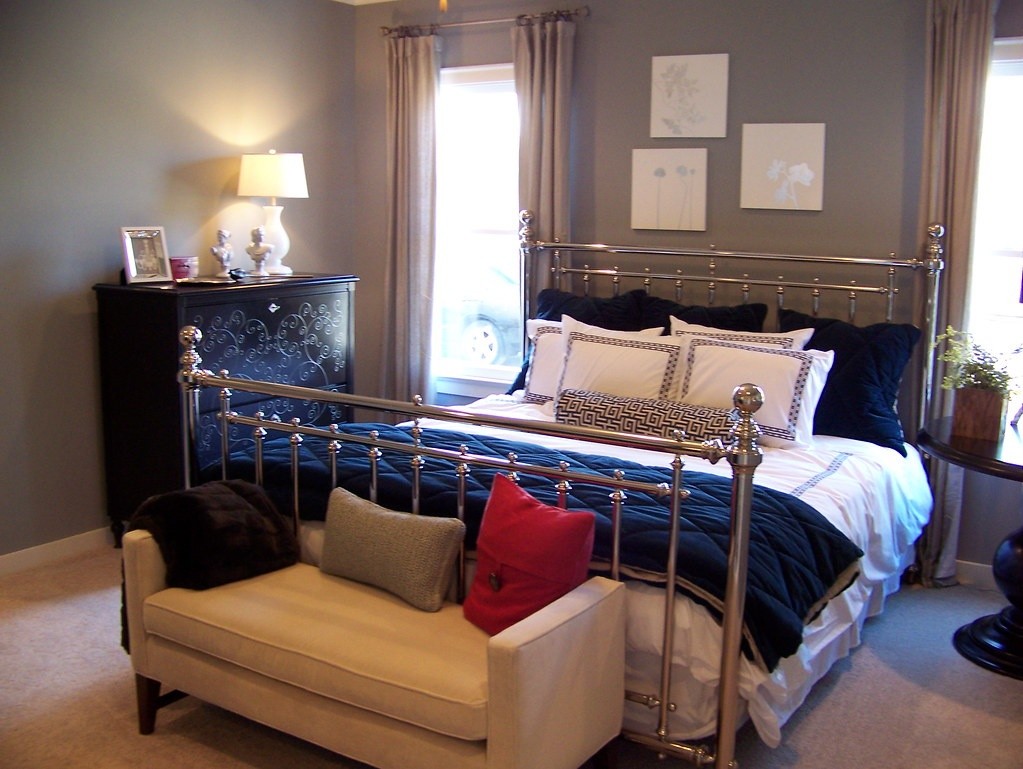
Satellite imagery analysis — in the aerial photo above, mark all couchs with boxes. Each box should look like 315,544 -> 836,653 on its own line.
122,514 -> 626,769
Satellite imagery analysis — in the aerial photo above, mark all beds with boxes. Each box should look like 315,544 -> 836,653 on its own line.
178,210 -> 945,769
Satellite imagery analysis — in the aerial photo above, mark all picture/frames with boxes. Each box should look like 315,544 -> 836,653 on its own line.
119,227 -> 173,284
649,54 -> 728,138
741,123 -> 825,211
631,148 -> 707,232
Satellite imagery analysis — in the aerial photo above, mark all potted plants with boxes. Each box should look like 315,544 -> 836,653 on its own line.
933,325 -> 1011,442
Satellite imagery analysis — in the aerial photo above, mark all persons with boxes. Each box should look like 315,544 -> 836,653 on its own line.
211,229 -> 232,278
246,225 -> 274,275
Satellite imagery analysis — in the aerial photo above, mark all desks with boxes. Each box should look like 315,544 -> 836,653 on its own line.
917,416 -> 1023,680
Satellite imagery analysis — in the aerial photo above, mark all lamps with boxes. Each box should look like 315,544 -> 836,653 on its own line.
237,149 -> 309,275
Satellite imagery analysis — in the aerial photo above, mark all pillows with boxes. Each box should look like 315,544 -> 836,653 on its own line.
779,308 -> 921,458
519,318 -> 664,405
675,333 -> 834,449
505,288 -> 647,394
320,487 -> 467,611
464,472 -> 597,636
554,389 -> 742,449
553,314 -> 680,402
669,315 -> 814,349
641,295 -> 768,336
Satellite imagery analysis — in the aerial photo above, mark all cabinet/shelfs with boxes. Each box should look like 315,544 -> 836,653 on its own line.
91,273 -> 360,548
